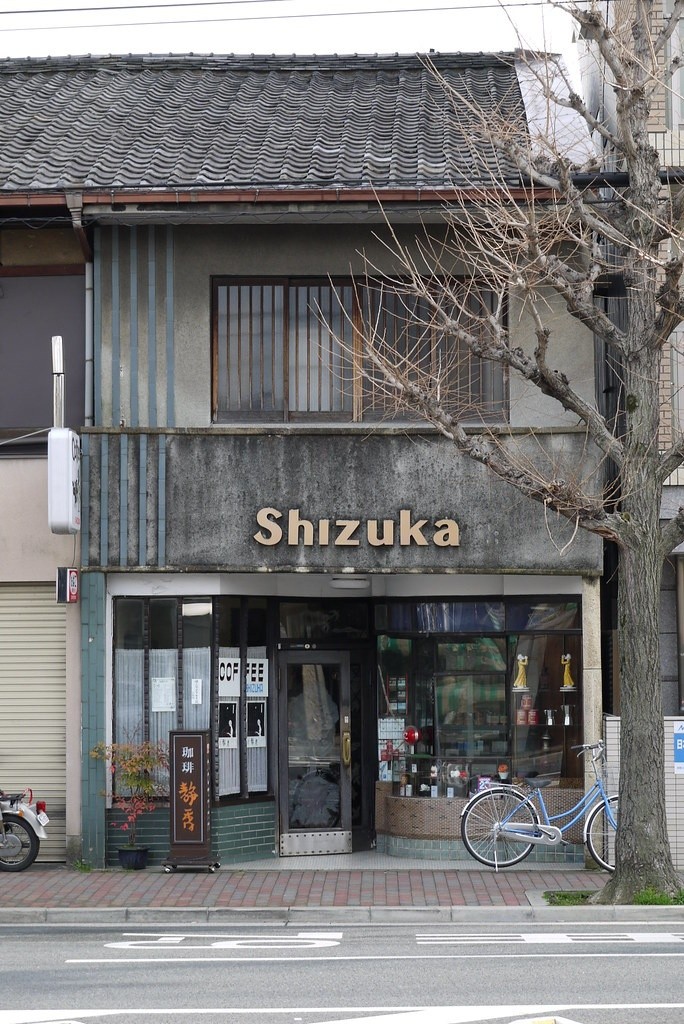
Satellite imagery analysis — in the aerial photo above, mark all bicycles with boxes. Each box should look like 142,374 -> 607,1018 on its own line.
458,740 -> 623,874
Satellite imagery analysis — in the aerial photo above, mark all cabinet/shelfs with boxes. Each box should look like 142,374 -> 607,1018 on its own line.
433,630 -> 584,784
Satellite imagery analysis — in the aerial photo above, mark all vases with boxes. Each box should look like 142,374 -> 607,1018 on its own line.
116,847 -> 150,871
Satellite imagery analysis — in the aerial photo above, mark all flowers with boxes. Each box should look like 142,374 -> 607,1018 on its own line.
89,740 -> 171,847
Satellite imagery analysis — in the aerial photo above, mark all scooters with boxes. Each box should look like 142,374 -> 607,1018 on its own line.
0,790 -> 49,872
